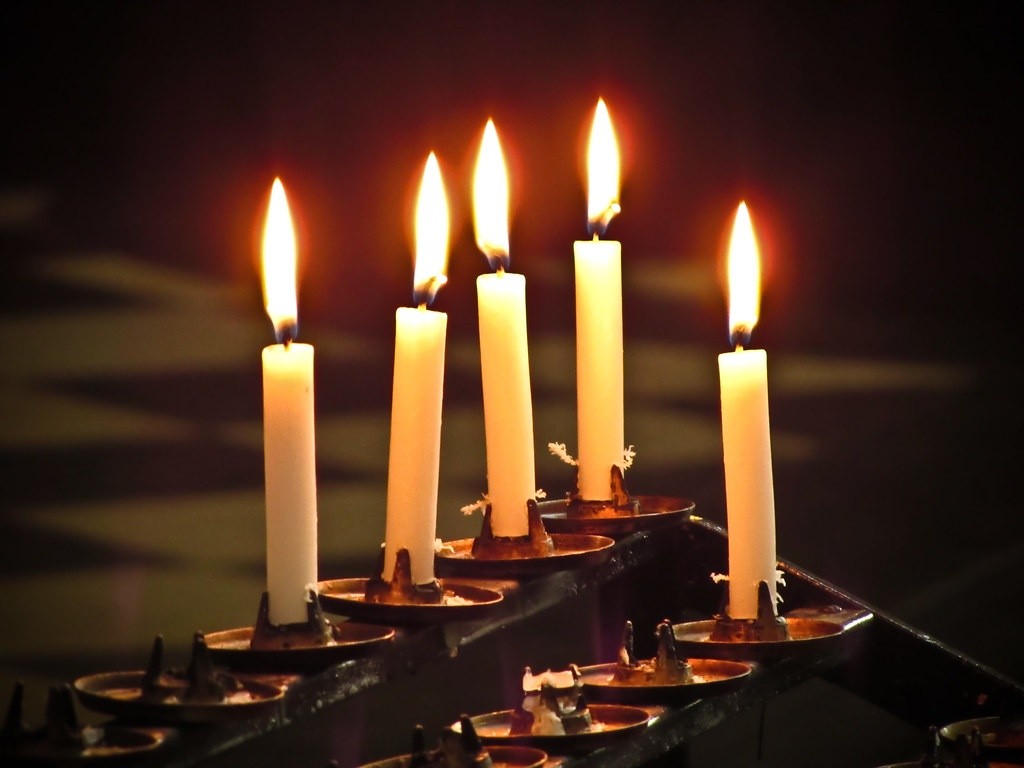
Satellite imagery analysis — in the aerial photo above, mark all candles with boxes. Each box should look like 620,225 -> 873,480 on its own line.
573,96 -> 625,500
471,117 -> 535,537
718,198 -> 778,618
260,175 -> 319,625
385,152 -> 450,585
